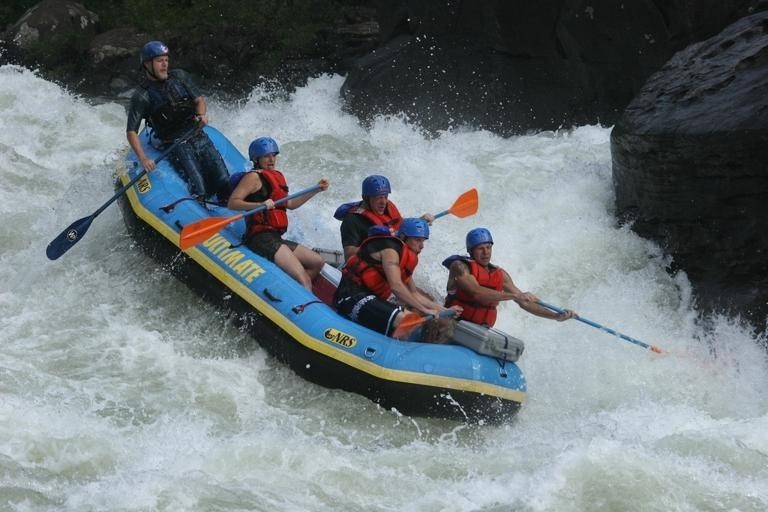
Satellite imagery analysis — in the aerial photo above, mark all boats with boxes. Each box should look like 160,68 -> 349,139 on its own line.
112,119 -> 528,428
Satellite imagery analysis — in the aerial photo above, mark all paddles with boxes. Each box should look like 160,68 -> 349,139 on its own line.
46,123 -> 205,261
423,189 -> 478,218
179,183 -> 323,251
393,309 -> 456,339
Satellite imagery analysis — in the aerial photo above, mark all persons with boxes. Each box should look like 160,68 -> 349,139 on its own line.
333,217 -> 464,338
227,137 -> 325,293
442,228 -> 575,328
126,40 -> 230,208
334,174 -> 434,261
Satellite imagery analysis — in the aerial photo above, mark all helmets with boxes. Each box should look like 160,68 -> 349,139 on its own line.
466,227 -> 493,252
248,137 -> 280,161
140,41 -> 169,63
397,219 -> 430,240
362,175 -> 391,199
